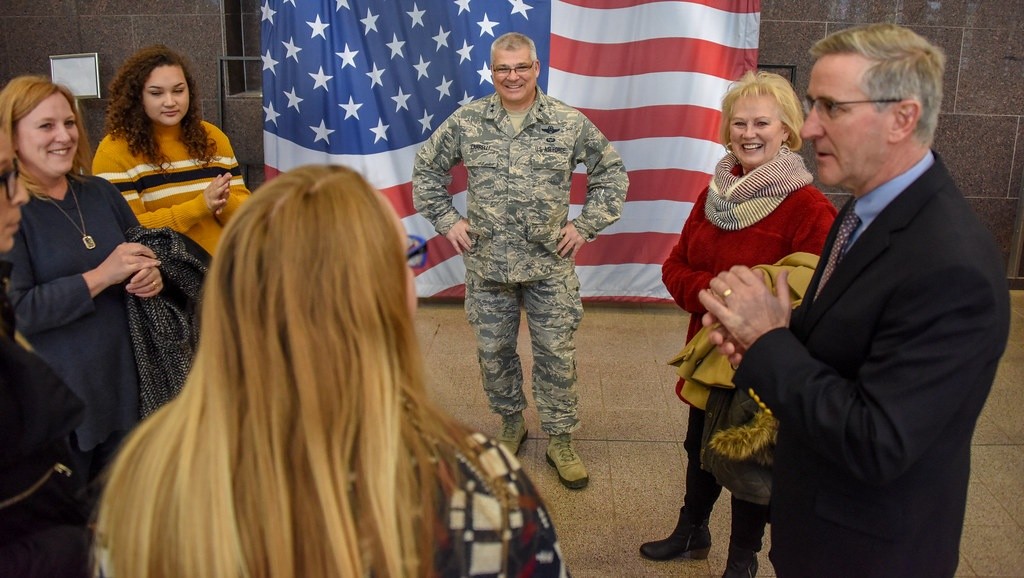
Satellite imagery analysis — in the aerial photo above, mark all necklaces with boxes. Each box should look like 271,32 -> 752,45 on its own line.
44,175 -> 96,249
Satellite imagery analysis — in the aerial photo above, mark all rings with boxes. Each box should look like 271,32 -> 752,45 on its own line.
723,289 -> 731,295
152,281 -> 159,288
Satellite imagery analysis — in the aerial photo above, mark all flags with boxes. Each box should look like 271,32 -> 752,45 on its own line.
262,0 -> 762,306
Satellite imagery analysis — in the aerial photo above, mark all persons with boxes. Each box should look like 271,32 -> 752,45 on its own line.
0,116 -> 126,578
87,166 -> 571,578
639,70 -> 839,578
698,21 -> 1012,578
0,73 -> 166,477
92,42 -> 253,260
412,34 -> 630,485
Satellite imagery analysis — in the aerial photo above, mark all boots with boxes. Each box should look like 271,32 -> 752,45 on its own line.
638,506 -> 712,561
722,542 -> 759,578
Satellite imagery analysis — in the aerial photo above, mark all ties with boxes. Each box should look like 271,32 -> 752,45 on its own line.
813,199 -> 861,303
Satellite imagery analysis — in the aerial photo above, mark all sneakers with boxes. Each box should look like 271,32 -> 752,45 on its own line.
546,433 -> 589,489
496,411 -> 528,457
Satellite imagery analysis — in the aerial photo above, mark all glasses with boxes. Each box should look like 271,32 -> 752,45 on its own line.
492,60 -> 536,77
803,94 -> 903,122
0,156 -> 21,200
